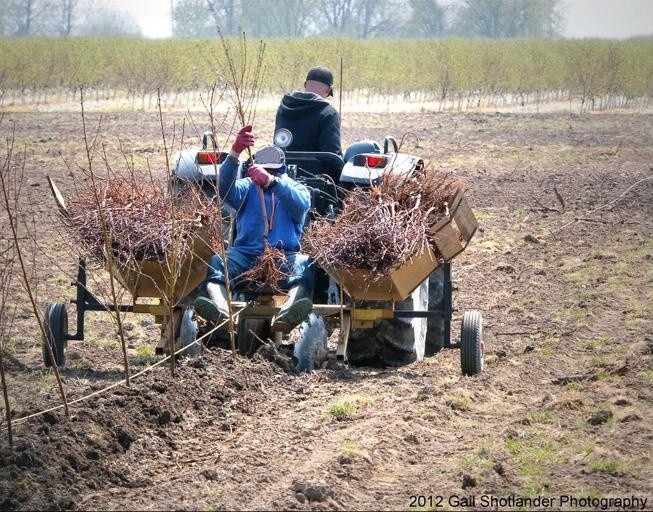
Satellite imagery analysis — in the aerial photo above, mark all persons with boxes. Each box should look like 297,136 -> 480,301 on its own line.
194,124 -> 314,332
274,66 -> 341,158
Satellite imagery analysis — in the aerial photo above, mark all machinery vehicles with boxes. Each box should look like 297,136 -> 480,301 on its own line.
36,130 -> 491,371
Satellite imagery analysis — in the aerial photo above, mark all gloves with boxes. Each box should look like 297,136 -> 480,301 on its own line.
253,143 -> 286,169
229,125 -> 254,159
247,165 -> 273,188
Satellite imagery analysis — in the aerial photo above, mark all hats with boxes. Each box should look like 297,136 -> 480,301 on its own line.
306,66 -> 333,98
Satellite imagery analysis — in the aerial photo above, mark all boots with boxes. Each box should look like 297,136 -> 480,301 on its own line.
193,280 -> 236,330
271,284 -> 312,332
